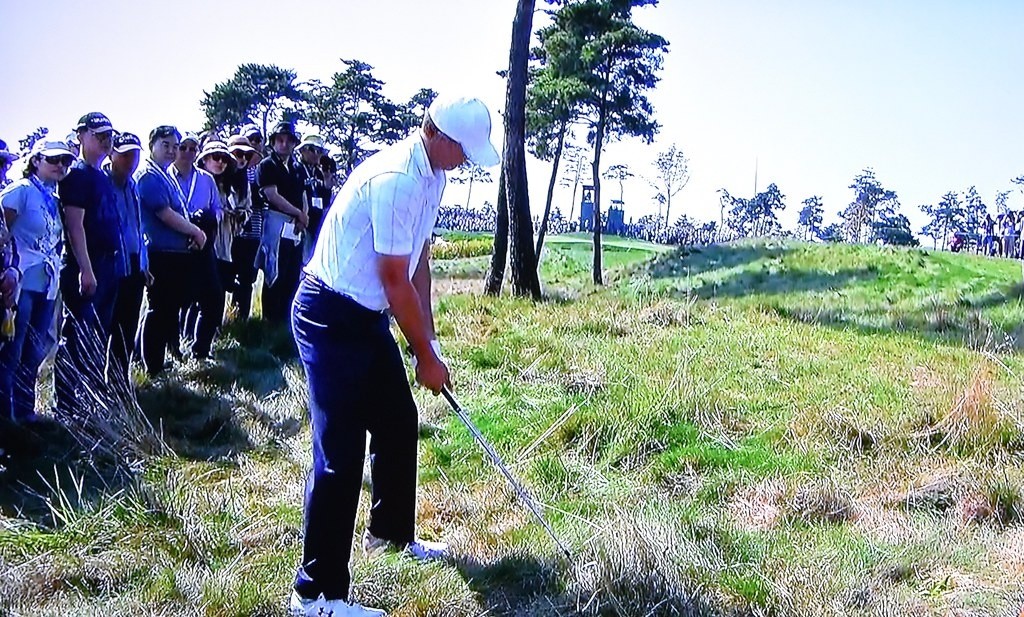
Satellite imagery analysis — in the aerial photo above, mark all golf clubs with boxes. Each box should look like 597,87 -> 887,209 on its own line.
404,341 -> 581,572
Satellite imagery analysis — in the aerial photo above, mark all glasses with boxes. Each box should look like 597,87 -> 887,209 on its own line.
213,153 -> 230,163
91,132 -> 111,140
178,144 -> 195,152
42,155 -> 72,166
308,146 -> 323,154
236,150 -> 253,161
249,137 -> 262,143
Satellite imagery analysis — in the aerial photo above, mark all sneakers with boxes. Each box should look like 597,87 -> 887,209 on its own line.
363,528 -> 450,562
289,592 -> 387,617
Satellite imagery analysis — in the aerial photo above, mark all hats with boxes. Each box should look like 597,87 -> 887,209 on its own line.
77,112 -> 120,134
0,140 -> 19,161
180,131 -> 199,144
227,135 -> 256,152
196,140 -> 238,165
32,136 -> 77,161
295,133 -> 326,150
264,121 -> 299,151
240,124 -> 263,138
429,92 -> 500,166
113,132 -> 141,152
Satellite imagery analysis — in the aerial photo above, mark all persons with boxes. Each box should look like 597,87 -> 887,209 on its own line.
0,111 -> 334,476
290,90 -> 501,617
